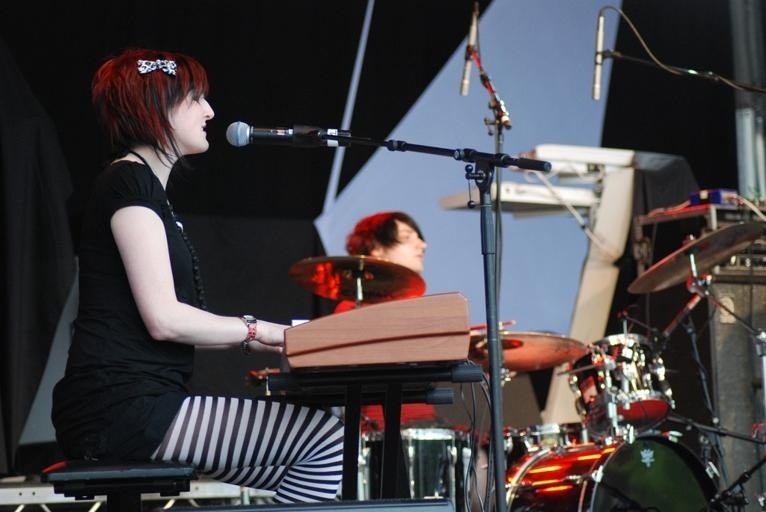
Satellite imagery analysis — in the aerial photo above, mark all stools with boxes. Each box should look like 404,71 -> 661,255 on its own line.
46,463 -> 194,512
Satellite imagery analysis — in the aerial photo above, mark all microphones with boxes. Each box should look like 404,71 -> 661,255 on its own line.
225,121 -> 352,148
460,12 -> 478,97
592,16 -> 605,100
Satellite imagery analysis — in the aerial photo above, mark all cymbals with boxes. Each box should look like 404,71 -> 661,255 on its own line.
628,222 -> 764,294
471,332 -> 585,372
292,255 -> 426,304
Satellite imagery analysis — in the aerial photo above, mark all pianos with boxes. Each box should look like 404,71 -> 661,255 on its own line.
283,292 -> 470,368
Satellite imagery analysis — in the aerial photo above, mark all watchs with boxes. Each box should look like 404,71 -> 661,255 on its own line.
242,313 -> 257,343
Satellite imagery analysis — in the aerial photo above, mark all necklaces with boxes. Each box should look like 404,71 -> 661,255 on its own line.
128,150 -> 209,311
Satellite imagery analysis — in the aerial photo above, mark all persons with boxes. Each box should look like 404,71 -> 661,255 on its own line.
333,211 -> 524,511
50,48 -> 345,504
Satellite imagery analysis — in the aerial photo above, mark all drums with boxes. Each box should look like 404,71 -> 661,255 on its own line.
590,419 -> 731,510
511,421 -> 582,459
357,426 -> 471,501
568,333 -> 676,437
505,426 -> 729,512
